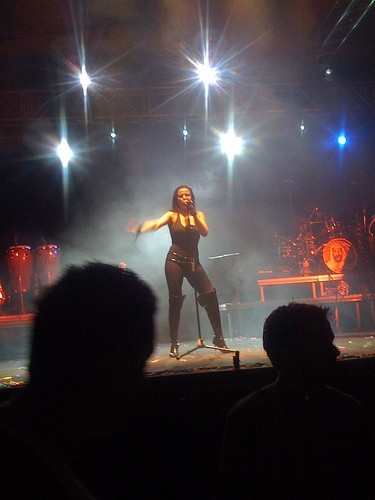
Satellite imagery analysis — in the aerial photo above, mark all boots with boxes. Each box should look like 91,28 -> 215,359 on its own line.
167,295 -> 185,358
198,289 -> 231,354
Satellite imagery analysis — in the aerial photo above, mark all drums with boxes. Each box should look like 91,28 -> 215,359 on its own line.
5,244 -> 34,294
32,244 -> 62,290
280,230 -> 358,274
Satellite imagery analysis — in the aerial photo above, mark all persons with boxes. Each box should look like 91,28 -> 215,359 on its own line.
223,303 -> 375,500
126,185 -> 229,357
0,263 -> 219,500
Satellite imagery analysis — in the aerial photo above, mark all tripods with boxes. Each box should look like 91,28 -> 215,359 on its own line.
176,204 -> 239,360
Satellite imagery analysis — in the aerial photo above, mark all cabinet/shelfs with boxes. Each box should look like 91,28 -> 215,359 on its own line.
216,273 -> 375,338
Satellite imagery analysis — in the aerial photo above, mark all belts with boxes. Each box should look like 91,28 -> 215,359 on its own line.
166,251 -> 199,265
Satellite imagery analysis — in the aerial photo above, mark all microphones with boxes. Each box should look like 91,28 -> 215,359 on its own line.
184,200 -> 193,205
